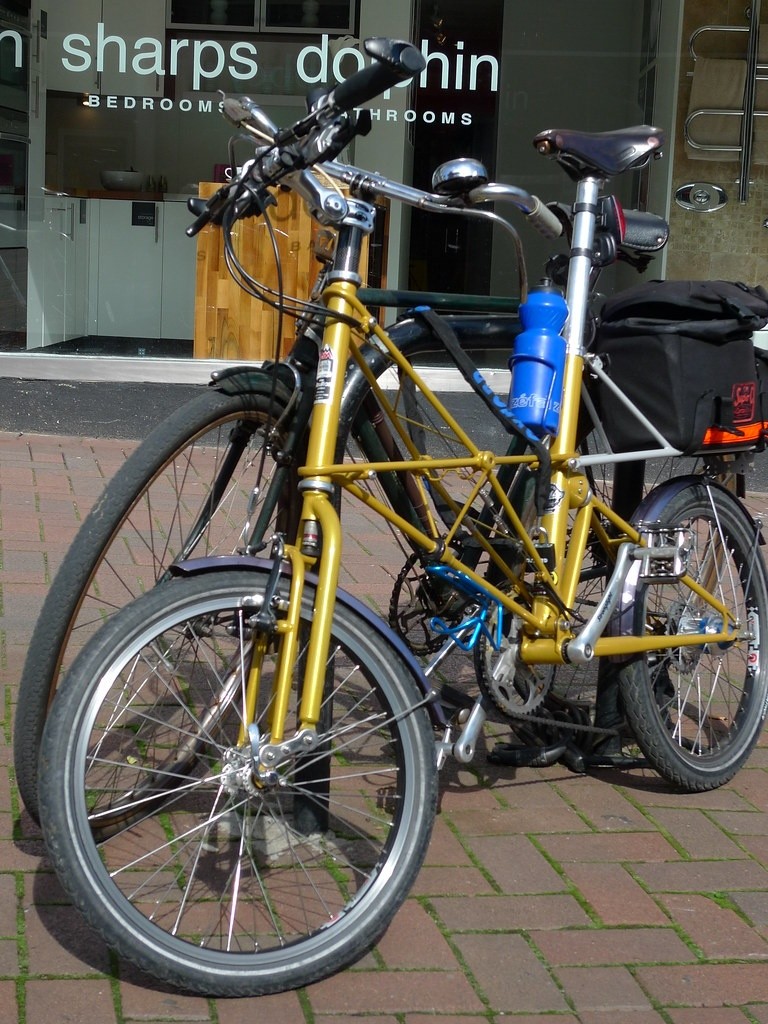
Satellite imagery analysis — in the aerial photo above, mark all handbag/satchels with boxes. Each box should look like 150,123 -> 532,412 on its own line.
588,272 -> 768,448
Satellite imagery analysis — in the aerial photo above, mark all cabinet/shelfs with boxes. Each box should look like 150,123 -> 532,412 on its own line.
191,181 -> 392,362
166,0 -> 356,35
43,194 -> 198,342
47,0 -> 167,100
0,0 -> 46,251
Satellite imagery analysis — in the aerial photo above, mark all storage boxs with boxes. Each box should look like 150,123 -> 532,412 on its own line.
595,277 -> 768,448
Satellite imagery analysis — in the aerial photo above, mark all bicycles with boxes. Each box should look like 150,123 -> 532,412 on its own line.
1,32 -> 766,998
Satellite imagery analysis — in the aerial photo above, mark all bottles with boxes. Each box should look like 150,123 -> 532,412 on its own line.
508,276 -> 568,433
